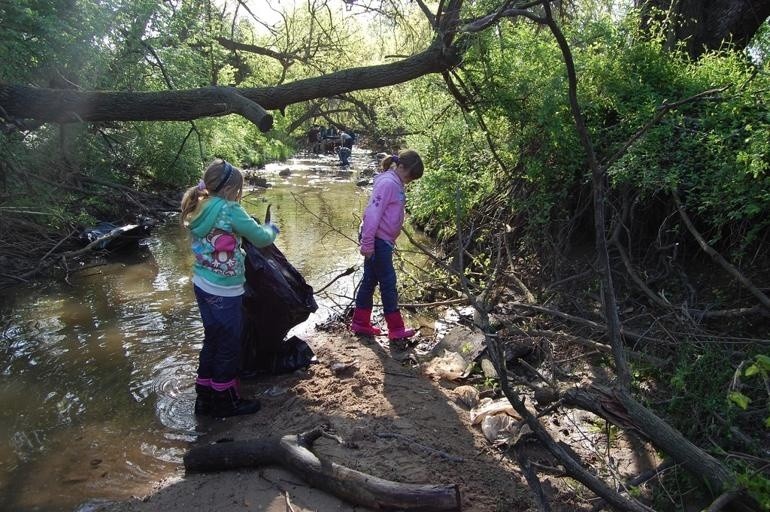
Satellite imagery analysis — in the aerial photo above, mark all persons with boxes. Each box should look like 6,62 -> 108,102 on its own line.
351,149 -> 423,341
180,160 -> 280,418
309,121 -> 355,166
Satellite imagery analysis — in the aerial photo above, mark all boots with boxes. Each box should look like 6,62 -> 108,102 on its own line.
384,309 -> 416,340
195,378 -> 261,419
351,307 -> 381,335
